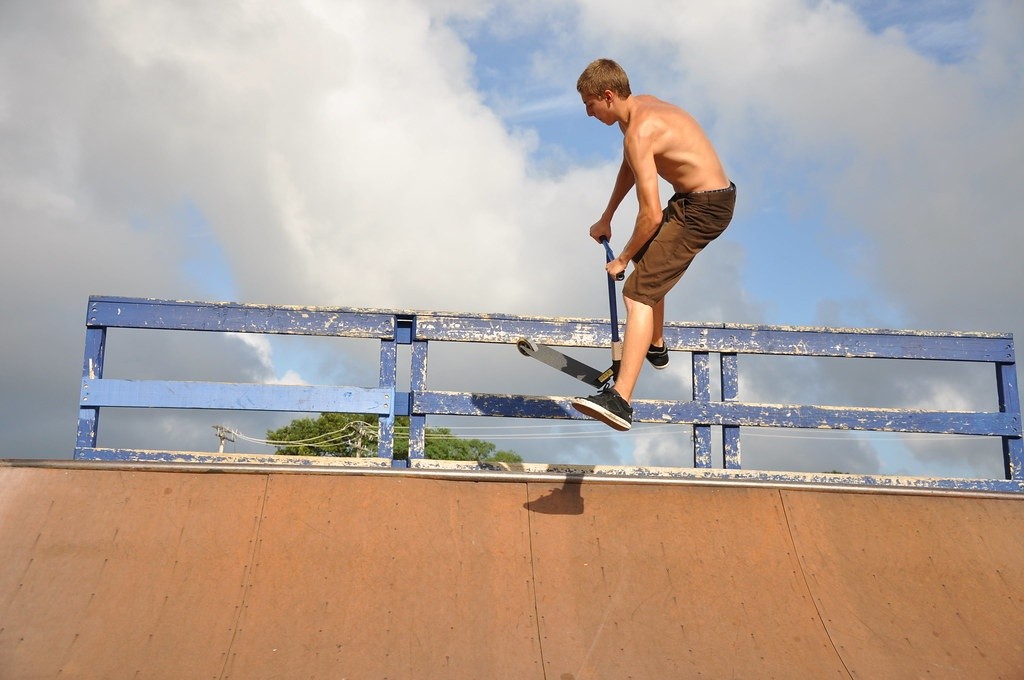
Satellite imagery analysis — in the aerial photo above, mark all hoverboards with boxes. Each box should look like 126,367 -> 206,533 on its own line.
516,236 -> 625,389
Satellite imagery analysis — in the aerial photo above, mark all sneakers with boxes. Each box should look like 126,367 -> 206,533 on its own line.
646,342 -> 669,369
572,384 -> 634,432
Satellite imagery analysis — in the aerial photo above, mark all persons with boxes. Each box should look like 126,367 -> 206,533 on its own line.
572,59 -> 737,430
